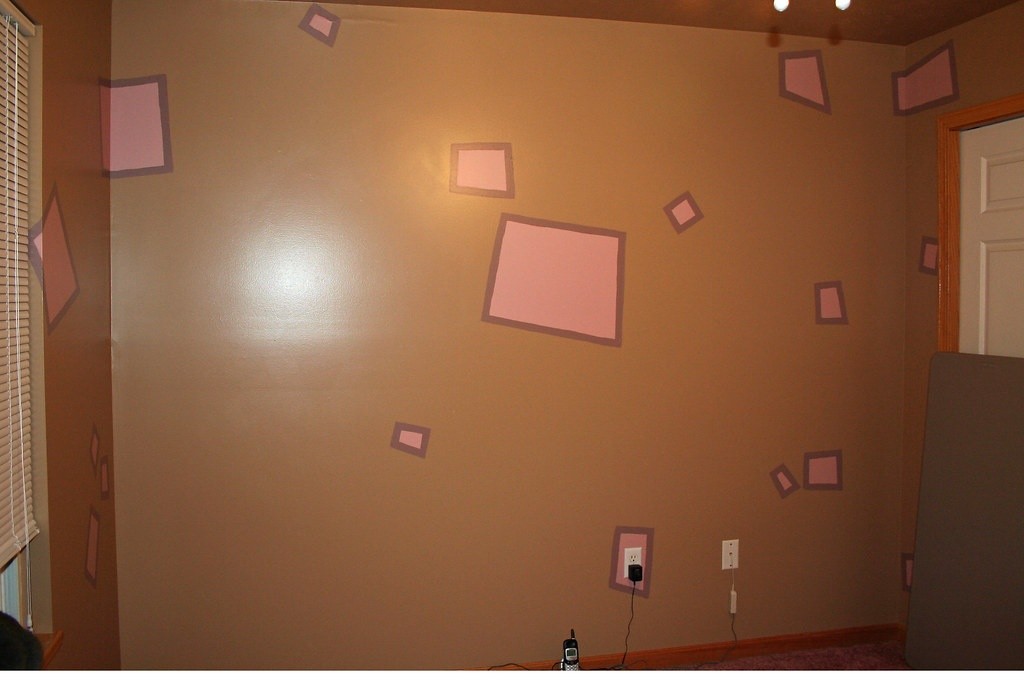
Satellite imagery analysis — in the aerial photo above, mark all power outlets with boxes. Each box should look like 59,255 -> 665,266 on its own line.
623,547 -> 642,577
722,539 -> 740,570
